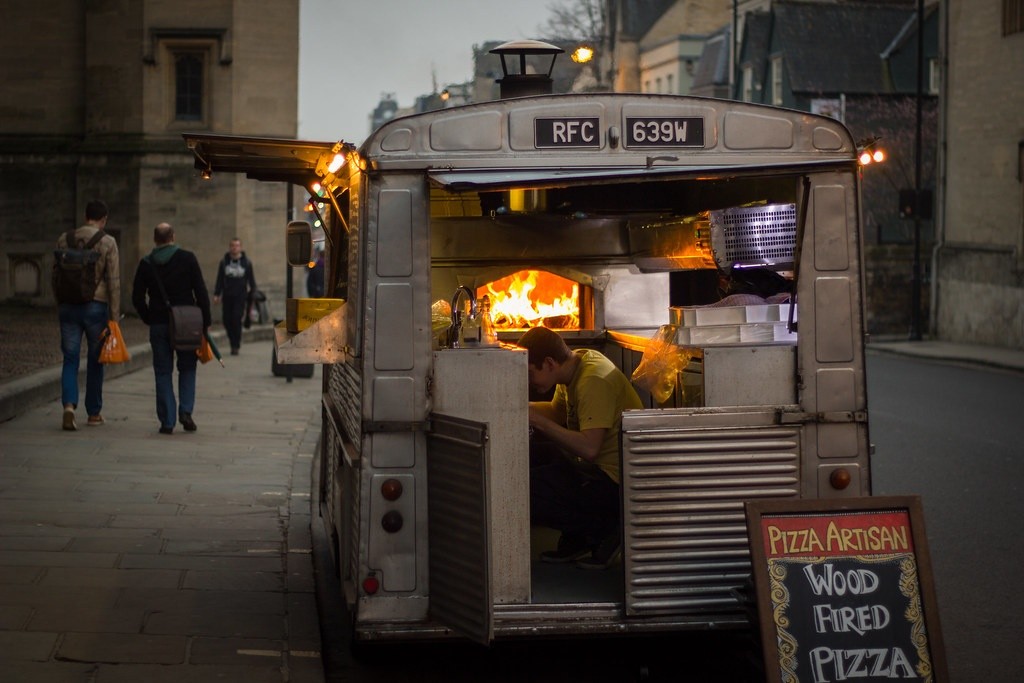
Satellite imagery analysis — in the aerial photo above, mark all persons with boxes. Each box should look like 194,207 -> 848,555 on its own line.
132,223 -> 213,433
214,236 -> 254,356
51,200 -> 125,431
518,327 -> 645,569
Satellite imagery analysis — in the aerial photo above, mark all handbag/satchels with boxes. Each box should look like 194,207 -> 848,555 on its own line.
195,335 -> 212,364
170,305 -> 202,350
631,325 -> 692,403
98,321 -> 131,363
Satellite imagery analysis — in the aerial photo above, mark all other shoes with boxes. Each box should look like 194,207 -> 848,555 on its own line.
88,414 -> 105,425
179,413 -> 197,430
62,403 -> 77,430
231,346 -> 238,354
159,429 -> 172,434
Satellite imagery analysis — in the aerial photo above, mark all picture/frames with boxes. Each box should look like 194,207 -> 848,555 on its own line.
745,494 -> 949,683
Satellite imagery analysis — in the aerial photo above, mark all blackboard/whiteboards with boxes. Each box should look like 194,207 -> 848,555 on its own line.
743,494 -> 950,683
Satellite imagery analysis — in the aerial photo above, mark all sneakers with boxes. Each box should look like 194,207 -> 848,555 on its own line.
541,525 -> 592,562
577,521 -> 621,570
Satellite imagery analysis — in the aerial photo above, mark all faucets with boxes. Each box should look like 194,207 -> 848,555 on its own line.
446,286 -> 477,350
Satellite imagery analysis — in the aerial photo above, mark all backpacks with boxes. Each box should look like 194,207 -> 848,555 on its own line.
50,230 -> 108,305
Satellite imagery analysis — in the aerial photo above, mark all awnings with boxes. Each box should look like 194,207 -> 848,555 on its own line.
180,130 -> 356,243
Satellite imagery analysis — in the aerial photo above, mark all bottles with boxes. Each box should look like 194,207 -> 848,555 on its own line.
464,314 -> 479,348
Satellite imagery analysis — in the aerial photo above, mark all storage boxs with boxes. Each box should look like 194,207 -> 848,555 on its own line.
595,269 -> 672,329
285,297 -> 344,333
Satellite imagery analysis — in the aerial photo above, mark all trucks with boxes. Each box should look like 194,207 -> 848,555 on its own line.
180,93 -> 877,683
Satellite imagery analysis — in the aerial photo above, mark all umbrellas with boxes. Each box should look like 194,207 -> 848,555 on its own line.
204,331 -> 225,369
